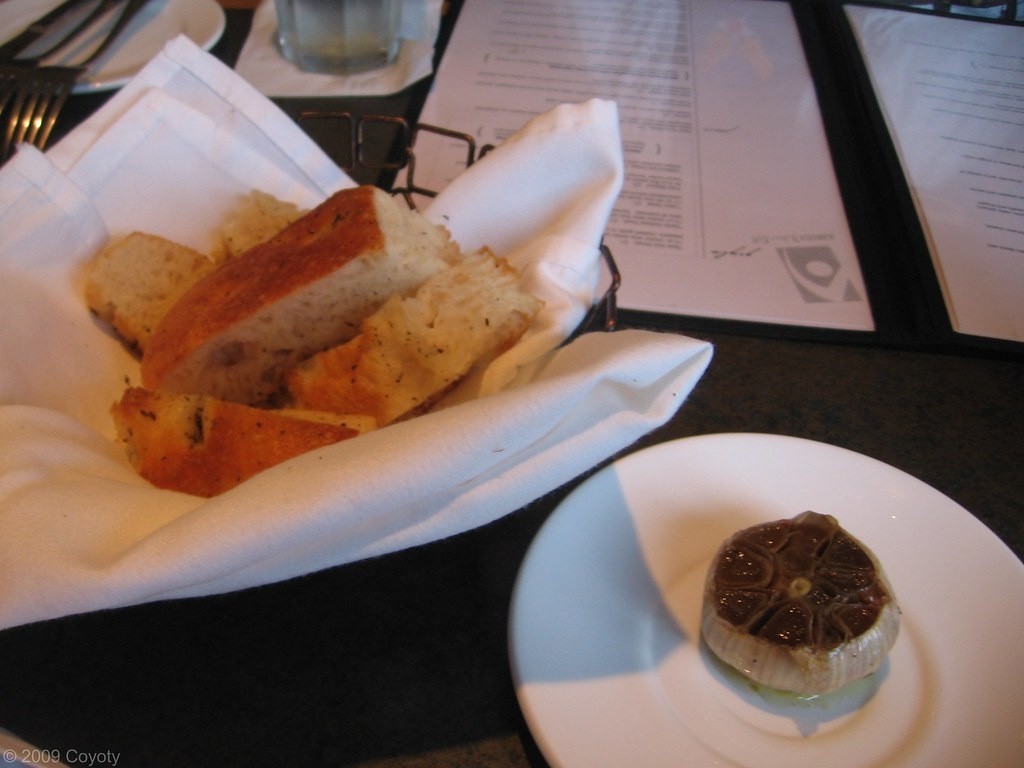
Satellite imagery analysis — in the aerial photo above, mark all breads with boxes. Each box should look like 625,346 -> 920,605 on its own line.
85,178 -> 544,496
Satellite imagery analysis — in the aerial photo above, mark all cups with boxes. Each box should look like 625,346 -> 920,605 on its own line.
274,0 -> 404,77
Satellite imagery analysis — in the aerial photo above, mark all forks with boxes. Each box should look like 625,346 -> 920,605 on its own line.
0,1 -> 151,161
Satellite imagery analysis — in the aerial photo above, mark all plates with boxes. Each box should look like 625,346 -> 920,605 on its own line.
506,430 -> 1024,768
0,0 -> 227,95
